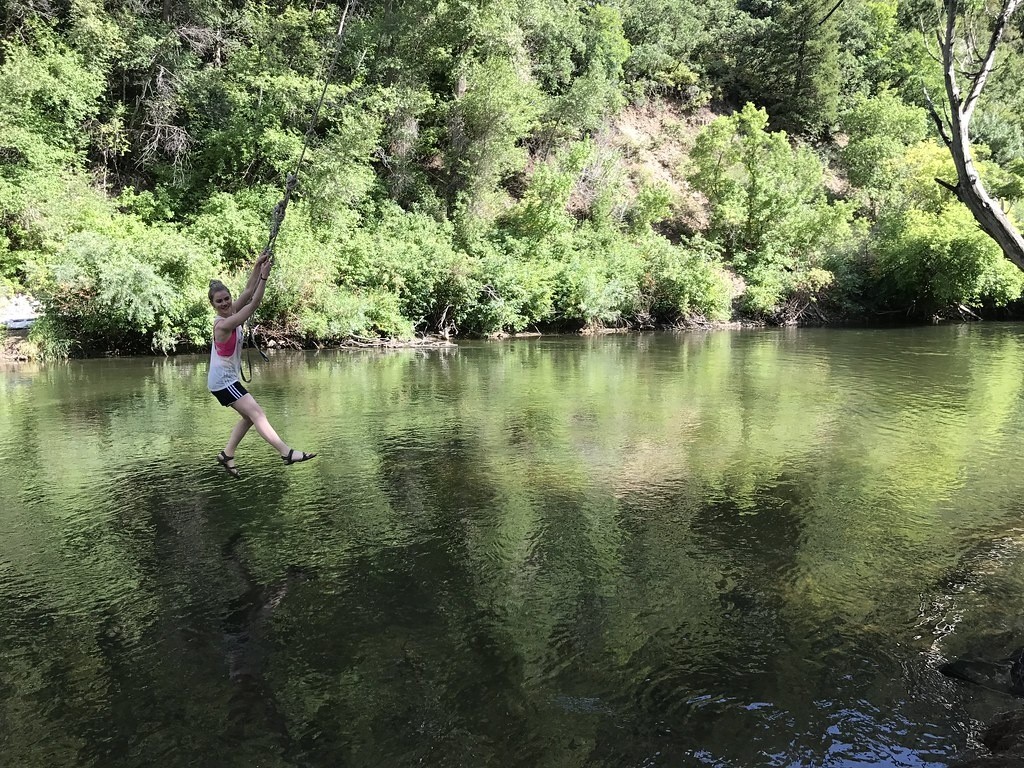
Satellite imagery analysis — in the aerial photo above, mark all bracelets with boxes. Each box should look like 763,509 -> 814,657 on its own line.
261,277 -> 267,280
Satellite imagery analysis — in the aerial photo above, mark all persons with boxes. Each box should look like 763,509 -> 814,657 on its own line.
202,250 -> 319,480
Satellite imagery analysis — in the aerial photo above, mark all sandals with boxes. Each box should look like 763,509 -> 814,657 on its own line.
216,451 -> 242,480
281,450 -> 317,465
